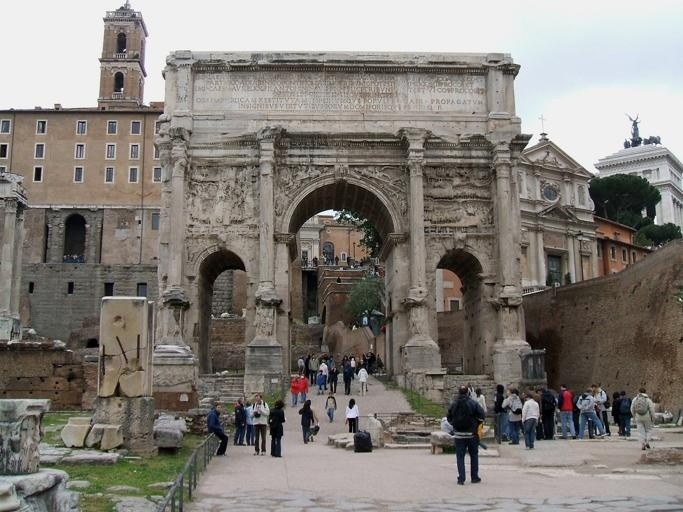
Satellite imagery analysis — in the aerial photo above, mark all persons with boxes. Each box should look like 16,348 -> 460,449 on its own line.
233,394 -> 285,457
441,417 -> 454,435
207,404 -> 229,458
631,120 -> 639,138
445,385 -> 486,484
630,388 -> 656,450
304,253 -> 363,268
298,352 -> 384,396
291,374 -> 309,407
337,275 -> 341,283
493,384 -> 633,449
299,399 -> 318,444
345,398 -> 359,433
468,386 -> 486,412
325,392 -> 337,423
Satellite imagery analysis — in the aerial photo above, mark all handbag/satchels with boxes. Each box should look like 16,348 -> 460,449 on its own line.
354,431 -> 372,452
634,397 -> 648,415
603,400 -> 611,409
620,399 -> 629,414
